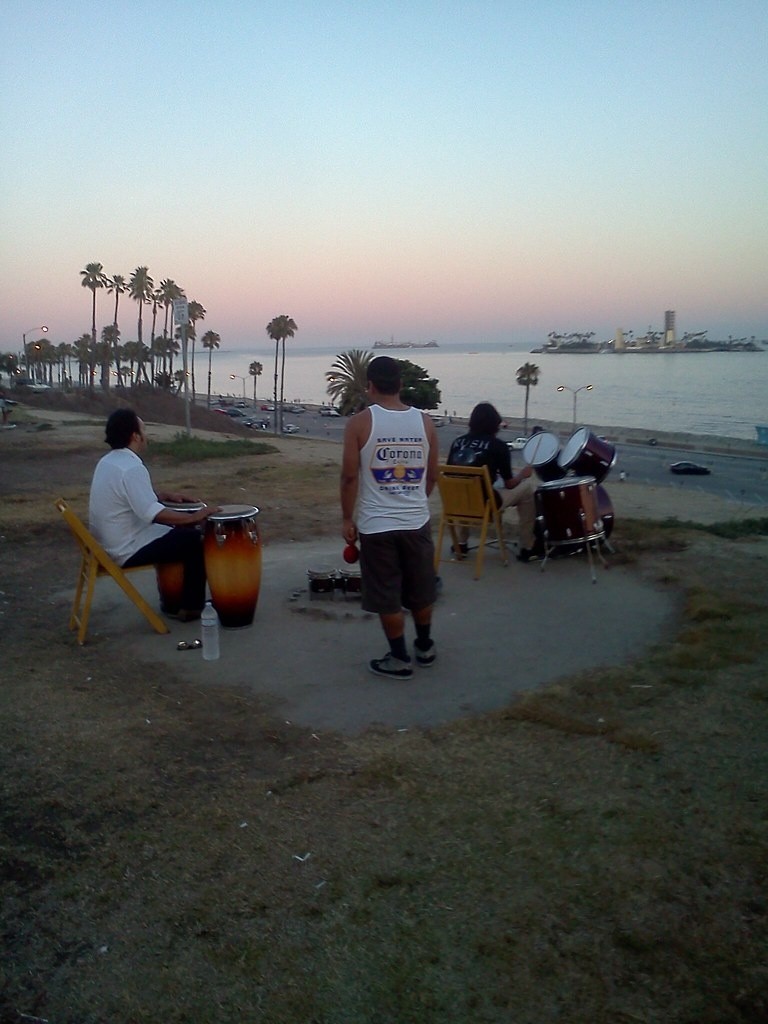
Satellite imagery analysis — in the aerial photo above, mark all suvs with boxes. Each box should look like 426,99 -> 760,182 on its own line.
319,407 -> 340,416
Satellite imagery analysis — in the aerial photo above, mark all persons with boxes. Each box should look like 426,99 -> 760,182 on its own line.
620,470 -> 625,482
444,401 -> 558,563
341,357 -> 439,679
445,409 -> 456,416
89,409 -> 224,623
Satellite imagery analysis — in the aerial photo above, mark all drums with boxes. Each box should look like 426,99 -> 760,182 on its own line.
521,431 -> 569,483
307,565 -> 334,594
536,477 -> 606,545
202,503 -> 261,629
154,499 -> 206,622
338,564 -> 362,594
596,484 -> 615,538
557,426 -> 618,484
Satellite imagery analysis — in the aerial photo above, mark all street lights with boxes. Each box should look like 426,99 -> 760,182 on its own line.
231,375 -> 252,410
23,328 -> 48,379
557,386 -> 594,432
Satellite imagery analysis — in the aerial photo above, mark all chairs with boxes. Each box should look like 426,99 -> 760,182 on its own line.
59,497 -> 170,646
433,463 -> 508,579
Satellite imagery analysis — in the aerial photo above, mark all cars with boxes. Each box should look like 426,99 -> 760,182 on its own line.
506,437 -> 528,450
209,398 -> 248,408
432,417 -> 443,427
260,403 -> 305,413
214,408 -> 270,429
282,424 -> 300,433
671,461 -> 711,475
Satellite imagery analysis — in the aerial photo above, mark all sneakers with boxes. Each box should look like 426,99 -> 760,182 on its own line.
412,637 -> 437,668
369,651 -> 414,680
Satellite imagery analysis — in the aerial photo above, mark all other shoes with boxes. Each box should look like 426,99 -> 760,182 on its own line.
178,599 -> 214,623
450,543 -> 469,557
3,423 -> 17,430
520,547 -> 552,563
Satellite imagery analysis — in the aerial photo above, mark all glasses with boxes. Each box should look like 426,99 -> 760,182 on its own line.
177,638 -> 204,651
